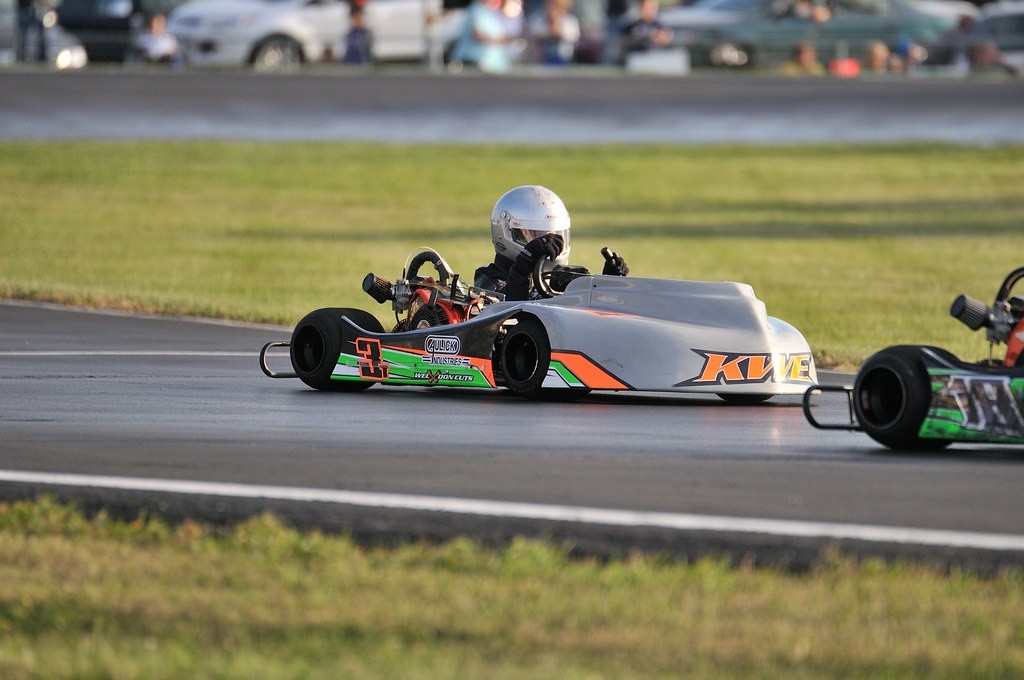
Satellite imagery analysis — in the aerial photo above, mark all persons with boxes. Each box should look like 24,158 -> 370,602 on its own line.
472,184 -> 629,302
13,0 -> 1024,78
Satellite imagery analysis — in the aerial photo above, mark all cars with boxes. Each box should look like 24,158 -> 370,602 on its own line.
168,0 -> 455,70
632,1 -> 1023,79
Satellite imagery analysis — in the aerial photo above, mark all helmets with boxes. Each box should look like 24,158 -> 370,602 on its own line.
491,186 -> 571,276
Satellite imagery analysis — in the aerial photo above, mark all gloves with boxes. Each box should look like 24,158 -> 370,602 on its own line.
516,234 -> 565,274
602,252 -> 629,276
